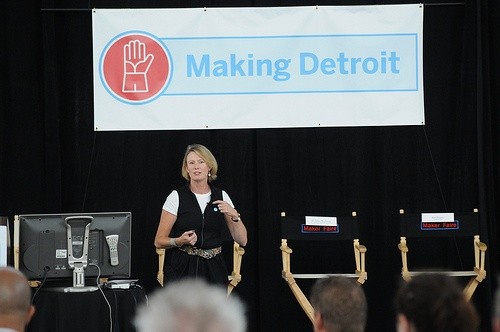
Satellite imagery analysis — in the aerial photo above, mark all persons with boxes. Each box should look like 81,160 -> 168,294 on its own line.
0,268 -> 35,332
395,272 -> 479,332
130,276 -> 249,332
154,144 -> 248,293
310,275 -> 368,332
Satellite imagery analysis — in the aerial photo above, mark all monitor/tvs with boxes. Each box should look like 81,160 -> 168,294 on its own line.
14,212 -> 132,292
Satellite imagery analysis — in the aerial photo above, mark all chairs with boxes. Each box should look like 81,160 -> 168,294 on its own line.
279,208 -> 368,331
400,208 -> 489,308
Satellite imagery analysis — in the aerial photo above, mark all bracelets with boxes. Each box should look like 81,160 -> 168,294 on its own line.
170,237 -> 182,247
231,213 -> 241,222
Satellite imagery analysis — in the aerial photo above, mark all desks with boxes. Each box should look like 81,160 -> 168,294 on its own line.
25,283 -> 146,332
